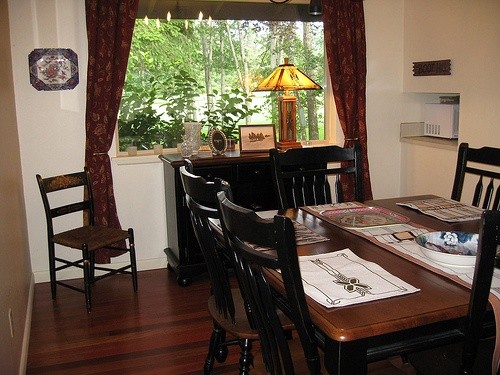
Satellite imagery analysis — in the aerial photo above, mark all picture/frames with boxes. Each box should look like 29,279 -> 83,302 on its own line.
238,124 -> 277,153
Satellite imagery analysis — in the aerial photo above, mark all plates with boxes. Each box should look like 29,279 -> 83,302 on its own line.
320,206 -> 410,229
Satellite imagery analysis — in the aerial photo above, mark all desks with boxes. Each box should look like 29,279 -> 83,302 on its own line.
208,194 -> 500,374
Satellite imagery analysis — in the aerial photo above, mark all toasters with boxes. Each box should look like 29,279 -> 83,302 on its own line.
423,103 -> 459,139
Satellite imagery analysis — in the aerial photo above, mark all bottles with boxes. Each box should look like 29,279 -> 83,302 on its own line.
185,122 -> 202,155
180,134 -> 193,157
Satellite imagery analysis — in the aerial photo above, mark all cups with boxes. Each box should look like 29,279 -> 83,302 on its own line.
227,139 -> 235,150
154,145 -> 163,155
177,143 -> 181,152
127,146 -> 137,156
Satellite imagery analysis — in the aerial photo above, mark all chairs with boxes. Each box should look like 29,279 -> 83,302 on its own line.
179,141 -> 500,375
36,166 -> 138,314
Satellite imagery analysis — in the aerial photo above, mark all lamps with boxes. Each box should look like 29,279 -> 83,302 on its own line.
269,0 -> 323,15
251,57 -> 324,150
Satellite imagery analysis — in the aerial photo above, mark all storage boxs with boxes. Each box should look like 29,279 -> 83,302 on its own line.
401,103 -> 460,139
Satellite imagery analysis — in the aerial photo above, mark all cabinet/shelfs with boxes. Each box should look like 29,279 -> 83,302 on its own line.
158,150 -> 326,287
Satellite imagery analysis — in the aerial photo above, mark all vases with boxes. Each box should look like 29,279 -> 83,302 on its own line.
183,122 -> 203,155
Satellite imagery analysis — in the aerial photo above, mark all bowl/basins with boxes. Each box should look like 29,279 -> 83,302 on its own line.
414,231 -> 479,267
